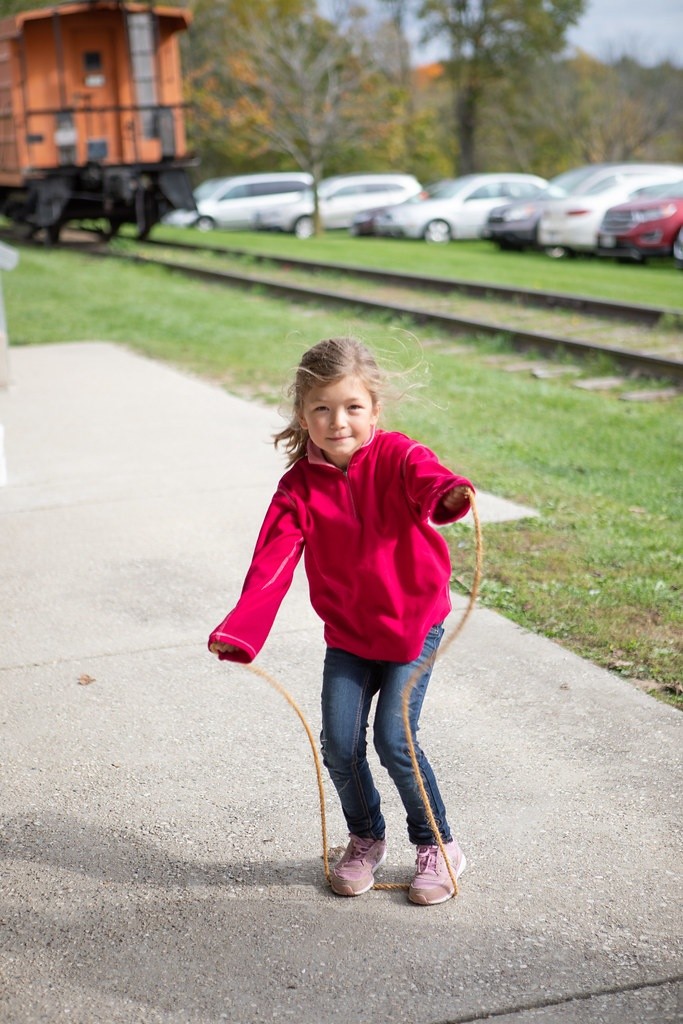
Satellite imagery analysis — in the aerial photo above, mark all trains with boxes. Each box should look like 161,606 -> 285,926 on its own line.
0,0 -> 201,248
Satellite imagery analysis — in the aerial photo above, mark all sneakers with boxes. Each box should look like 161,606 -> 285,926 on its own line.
330,833 -> 387,896
407,840 -> 467,905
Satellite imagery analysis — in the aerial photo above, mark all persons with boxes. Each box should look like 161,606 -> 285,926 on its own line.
208,336 -> 473,906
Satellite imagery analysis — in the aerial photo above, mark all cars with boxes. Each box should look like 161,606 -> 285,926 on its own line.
256,172 -> 421,238
161,169 -> 318,234
345,159 -> 683,269
